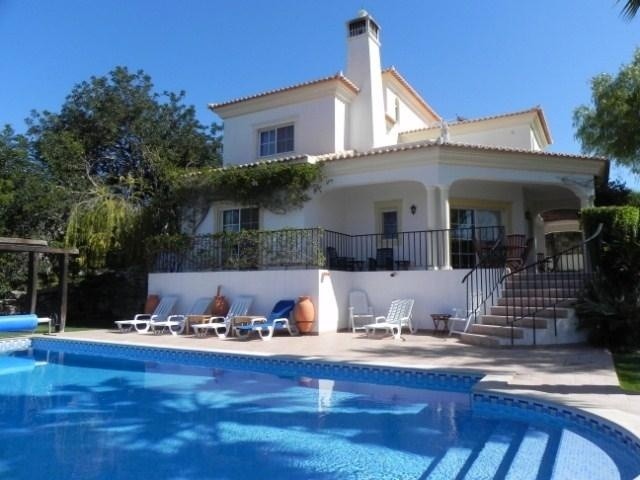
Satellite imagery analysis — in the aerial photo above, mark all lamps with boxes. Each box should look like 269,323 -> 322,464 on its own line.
410,204 -> 416,215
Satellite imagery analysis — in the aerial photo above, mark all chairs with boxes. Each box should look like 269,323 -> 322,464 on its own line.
191,296 -> 254,339
363,298 -> 416,340
474,238 -> 502,271
325,246 -> 348,269
447,291 -> 485,338
368,247 -> 395,271
232,299 -> 298,340
150,298 -> 213,335
115,295 -> 180,335
345,289 -> 377,334
508,232 -> 524,272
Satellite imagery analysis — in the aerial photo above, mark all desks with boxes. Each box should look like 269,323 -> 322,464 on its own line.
430,312 -> 452,336
233,313 -> 266,337
336,254 -> 369,272
394,258 -> 411,271
491,246 -> 526,270
185,315 -> 217,336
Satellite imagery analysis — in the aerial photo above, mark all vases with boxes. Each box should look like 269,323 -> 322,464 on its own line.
293,295 -> 315,334
213,295 -> 228,318
144,295 -> 160,315
